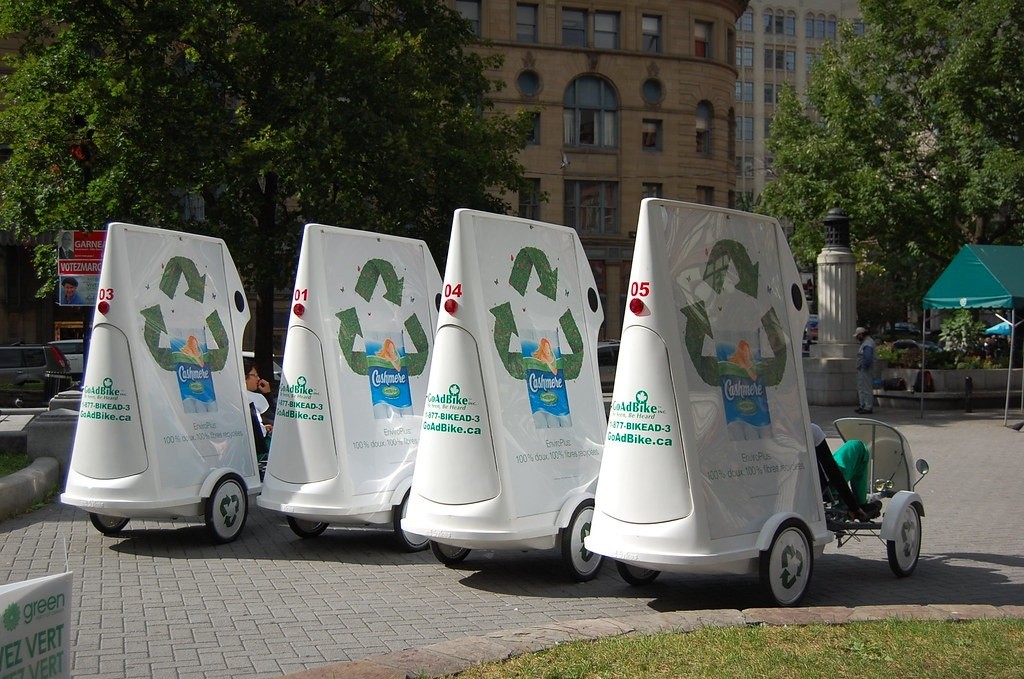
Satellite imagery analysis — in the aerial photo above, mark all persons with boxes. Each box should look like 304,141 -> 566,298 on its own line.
59,232 -> 74,259
854,327 -> 877,415
62,278 -> 83,304
244,363 -> 274,462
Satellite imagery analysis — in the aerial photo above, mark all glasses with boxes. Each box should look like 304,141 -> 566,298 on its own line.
247,373 -> 258,377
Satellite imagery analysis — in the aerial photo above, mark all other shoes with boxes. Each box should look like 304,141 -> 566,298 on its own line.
854,408 -> 861,412
858,501 -> 882,522
859,409 -> 872,414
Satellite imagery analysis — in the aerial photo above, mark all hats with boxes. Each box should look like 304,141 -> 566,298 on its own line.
853,327 -> 866,336
62,277 -> 78,287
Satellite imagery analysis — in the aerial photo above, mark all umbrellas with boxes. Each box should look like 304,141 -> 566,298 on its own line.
986,322 -> 1010,335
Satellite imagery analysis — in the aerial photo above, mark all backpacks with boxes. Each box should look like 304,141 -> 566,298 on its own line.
911,371 -> 934,394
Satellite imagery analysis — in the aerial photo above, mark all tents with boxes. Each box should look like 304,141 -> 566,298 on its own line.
921,245 -> 1024,425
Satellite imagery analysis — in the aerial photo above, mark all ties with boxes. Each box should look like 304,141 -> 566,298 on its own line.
65,251 -> 69,258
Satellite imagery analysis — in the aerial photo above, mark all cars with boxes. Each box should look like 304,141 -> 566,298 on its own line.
884,321 -> 933,340
802,313 -> 819,340
242,351 -> 282,384
597,339 -> 621,394
0,337 -> 86,407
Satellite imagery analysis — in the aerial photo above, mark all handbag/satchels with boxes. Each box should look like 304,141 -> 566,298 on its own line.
884,377 -> 907,391
